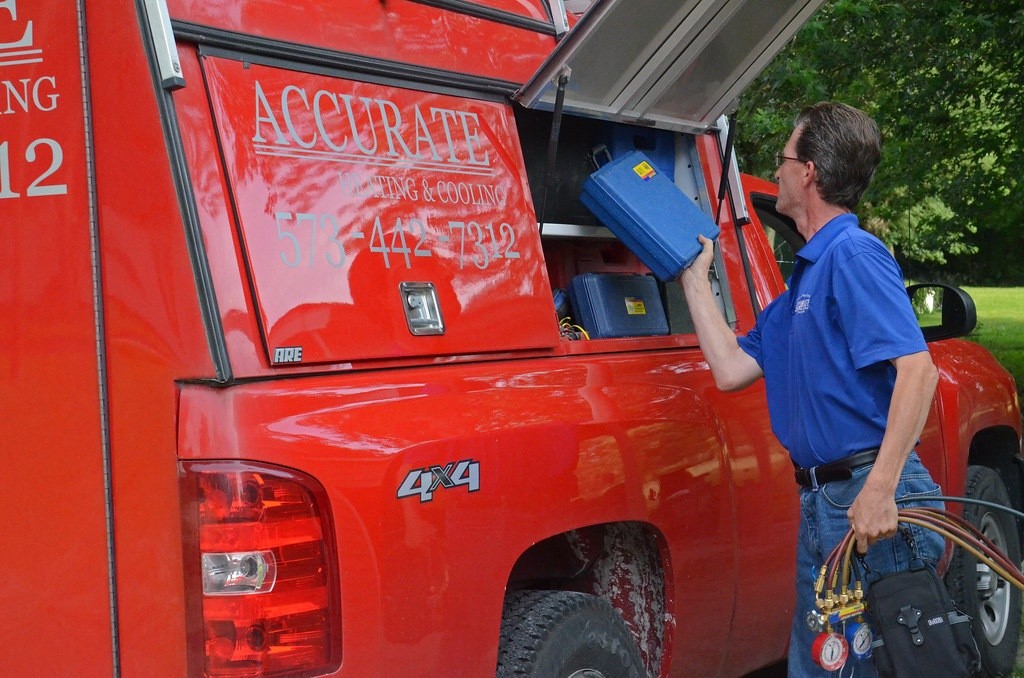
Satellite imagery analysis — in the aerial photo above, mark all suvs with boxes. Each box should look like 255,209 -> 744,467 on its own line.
0,0 -> 1024,678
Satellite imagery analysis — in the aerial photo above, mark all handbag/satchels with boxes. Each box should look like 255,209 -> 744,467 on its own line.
853,522 -> 984,678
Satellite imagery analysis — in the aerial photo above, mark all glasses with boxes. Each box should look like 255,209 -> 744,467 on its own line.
774,151 -> 809,167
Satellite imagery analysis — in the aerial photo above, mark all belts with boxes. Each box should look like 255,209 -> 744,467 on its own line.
795,449 -> 881,489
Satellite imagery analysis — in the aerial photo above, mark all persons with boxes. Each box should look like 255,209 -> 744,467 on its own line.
680,101 -> 948,678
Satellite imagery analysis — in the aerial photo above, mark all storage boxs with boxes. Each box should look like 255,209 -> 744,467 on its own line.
571,273 -> 669,341
580,151 -> 720,284
